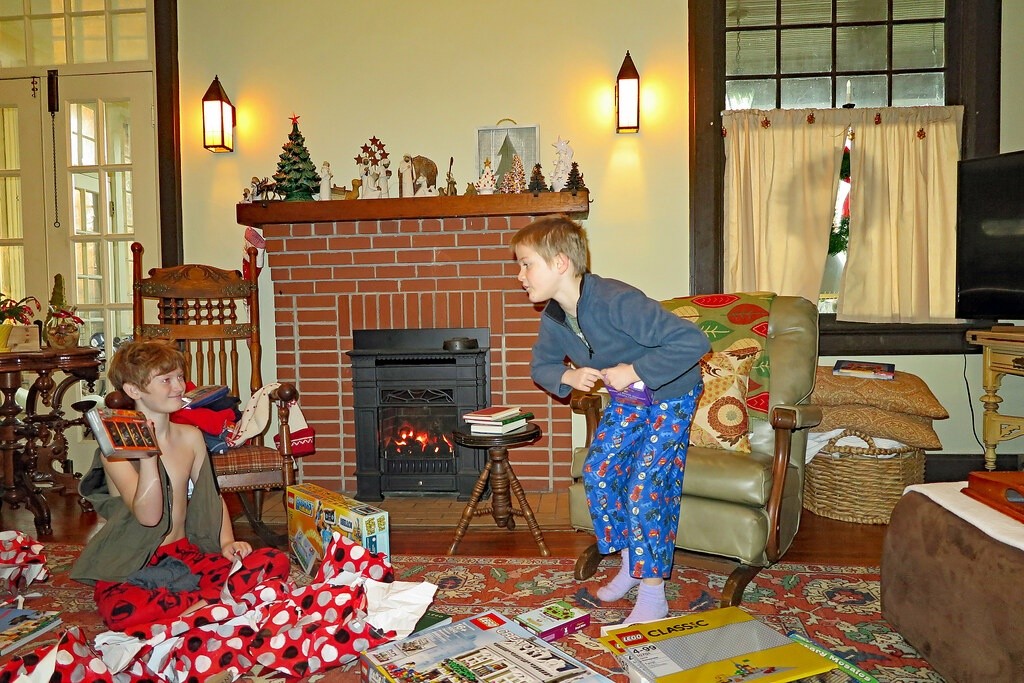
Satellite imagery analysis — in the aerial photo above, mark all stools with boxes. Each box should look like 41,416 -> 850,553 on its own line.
440,423 -> 549,559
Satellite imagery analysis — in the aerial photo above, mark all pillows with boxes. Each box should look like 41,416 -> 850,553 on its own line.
807,364 -> 951,418
688,345 -> 758,452
808,400 -> 942,450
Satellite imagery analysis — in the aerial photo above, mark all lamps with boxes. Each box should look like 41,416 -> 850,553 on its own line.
615,50 -> 640,134
202,75 -> 236,153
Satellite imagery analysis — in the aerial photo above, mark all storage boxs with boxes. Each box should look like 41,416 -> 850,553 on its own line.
285,483 -> 391,577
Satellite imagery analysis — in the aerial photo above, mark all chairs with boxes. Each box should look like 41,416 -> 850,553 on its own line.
127,242 -> 299,552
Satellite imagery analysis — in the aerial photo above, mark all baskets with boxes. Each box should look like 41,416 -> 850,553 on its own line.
802,430 -> 926,523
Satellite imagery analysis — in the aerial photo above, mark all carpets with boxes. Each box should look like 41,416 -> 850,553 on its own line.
0,543 -> 945,683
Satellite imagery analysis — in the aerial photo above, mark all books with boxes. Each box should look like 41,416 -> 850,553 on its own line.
832,359 -> 895,380
463,405 -> 523,420
0,607 -> 64,657
465,411 -> 535,425
471,418 -> 528,434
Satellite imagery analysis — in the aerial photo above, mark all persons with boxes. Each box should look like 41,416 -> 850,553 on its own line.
69,331 -> 252,588
510,211 -> 711,623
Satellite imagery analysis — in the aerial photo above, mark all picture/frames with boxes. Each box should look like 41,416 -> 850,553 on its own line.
476,124 -> 541,190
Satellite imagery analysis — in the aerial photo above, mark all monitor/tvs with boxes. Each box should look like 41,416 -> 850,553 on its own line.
957,151 -> 1024,322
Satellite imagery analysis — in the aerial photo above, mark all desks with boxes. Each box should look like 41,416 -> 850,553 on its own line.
0,346 -> 103,533
966,331 -> 1024,469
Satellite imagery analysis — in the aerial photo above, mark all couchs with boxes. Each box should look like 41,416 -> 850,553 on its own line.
568,291 -> 821,608
